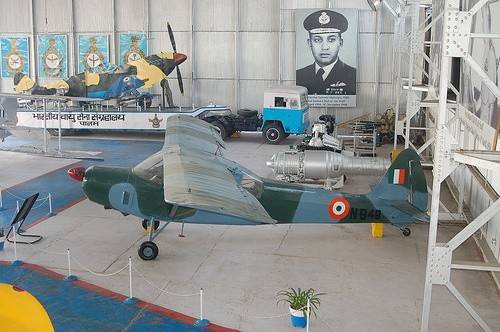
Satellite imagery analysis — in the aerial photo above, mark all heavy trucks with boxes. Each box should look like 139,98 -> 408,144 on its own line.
12,85 -> 310,143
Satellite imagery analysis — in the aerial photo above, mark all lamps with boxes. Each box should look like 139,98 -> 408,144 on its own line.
367,0 -> 399,19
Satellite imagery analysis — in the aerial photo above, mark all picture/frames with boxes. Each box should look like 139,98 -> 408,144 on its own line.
0,36 -> 30,80
78,34 -> 110,74
37,33 -> 69,80
119,32 -> 149,67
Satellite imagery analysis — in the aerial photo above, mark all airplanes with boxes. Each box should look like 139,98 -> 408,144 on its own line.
13,20 -> 189,95
68,113 -> 448,261
12,71 -> 152,111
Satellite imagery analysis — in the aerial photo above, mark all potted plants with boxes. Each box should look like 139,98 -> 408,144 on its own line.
276,288 -> 327,328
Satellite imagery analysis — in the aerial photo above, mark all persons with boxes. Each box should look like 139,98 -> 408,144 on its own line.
296,10 -> 356,95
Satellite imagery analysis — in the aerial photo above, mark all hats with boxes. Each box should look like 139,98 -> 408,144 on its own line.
303,10 -> 347,33
